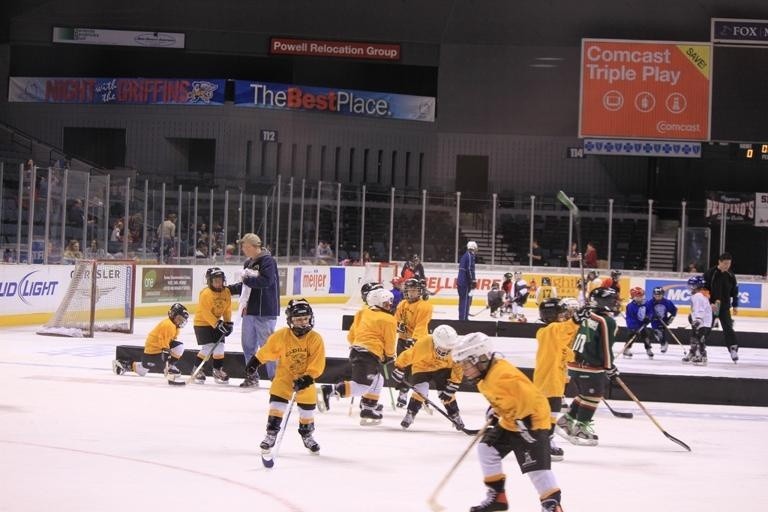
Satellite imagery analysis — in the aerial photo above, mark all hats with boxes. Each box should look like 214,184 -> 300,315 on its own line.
466,241 -> 478,252
235,232 -> 261,248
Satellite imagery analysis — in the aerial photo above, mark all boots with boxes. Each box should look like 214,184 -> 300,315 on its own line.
540,498 -> 563,512
469,476 -> 509,512
321,382 -> 345,411
448,410 -> 466,431
239,372 -> 259,387
556,412 -> 601,440
212,367 -> 229,381
190,365 -> 207,380
400,408 -> 416,428
357,396 -> 385,421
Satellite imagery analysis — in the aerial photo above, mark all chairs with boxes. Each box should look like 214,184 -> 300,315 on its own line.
2,170 -> 656,271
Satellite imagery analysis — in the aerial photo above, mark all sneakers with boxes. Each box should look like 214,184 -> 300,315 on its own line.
397,391 -> 408,405
622,341 -> 742,363
260,432 -> 277,449
546,434 -> 565,458
489,307 -> 527,324
115,360 -> 124,375
168,367 -> 181,375
301,435 -> 320,451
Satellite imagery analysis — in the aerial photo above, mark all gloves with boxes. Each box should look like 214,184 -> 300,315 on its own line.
471,280 -> 477,290
666,316 -> 674,326
438,381 -> 461,403
216,320 -> 233,337
391,366 -> 407,387
161,348 -> 172,364
604,365 -> 621,382
293,375 -> 313,391
643,318 -> 649,326
380,353 -> 397,364
513,414 -> 537,445
484,406 -> 499,425
396,323 -> 407,332
405,338 -> 418,350
572,307 -> 590,325
692,317 -> 703,329
243,355 -> 263,378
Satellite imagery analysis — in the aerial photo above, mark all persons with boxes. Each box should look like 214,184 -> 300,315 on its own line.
235,233 -> 280,389
622,286 -> 655,357
396,323 -> 465,429
109,304 -> 190,385
317,281 -> 396,421
18,154 -> 62,209
705,252 -> 739,362
194,268 -> 232,385
531,273 -> 621,448
391,252 -> 432,413
487,238 -> 624,321
454,240 -> 479,322
646,286 -> 678,352
451,332 -> 560,510
242,298 -> 325,451
683,276 -> 713,363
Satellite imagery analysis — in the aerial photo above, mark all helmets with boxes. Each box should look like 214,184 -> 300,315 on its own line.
687,275 -> 707,291
403,278 -> 428,304
405,254 -> 421,272
630,287 -> 646,301
652,286 -> 664,298
360,282 -> 395,312
206,267 -> 227,293
432,324 -> 457,360
490,269 -> 624,286
538,297 -> 570,323
451,331 -> 498,382
585,286 -> 621,315
167,302 -> 190,328
284,298 -> 315,339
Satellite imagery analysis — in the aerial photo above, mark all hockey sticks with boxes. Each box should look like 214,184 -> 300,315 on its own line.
402,380 -> 495,436
261,391 -> 296,468
556,191 -> 587,307
468,306 -> 490,317
618,376 -> 691,452
423,286 -> 441,295
168,333 -> 224,386
659,318 -> 687,355
600,398 -> 633,418
428,414 -> 494,512
